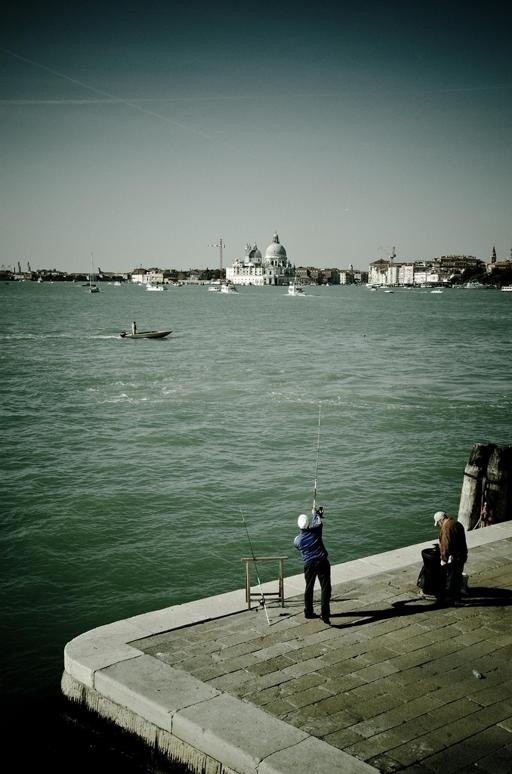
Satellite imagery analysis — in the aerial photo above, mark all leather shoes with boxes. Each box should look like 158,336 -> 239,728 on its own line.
305,612 -> 316,618
320,615 -> 331,624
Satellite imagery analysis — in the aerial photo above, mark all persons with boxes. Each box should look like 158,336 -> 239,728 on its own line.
433,511 -> 468,600
132,321 -> 136,335
293,505 -> 332,626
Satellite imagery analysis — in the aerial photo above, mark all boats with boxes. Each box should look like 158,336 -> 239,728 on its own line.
431,290 -> 443,294
478,284 -> 494,289
288,285 -> 306,297
384,290 -> 394,293
123,328 -> 173,338
82,283 -> 99,292
144,286 -> 168,292
501,287 -> 512,292
208,284 -> 240,295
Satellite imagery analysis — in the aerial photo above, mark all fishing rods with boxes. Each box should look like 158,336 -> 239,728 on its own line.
312,401 -> 325,527
239,506 -> 271,626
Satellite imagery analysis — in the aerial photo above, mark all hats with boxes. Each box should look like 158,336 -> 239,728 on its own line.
297,514 -> 309,530
433,512 -> 446,527
